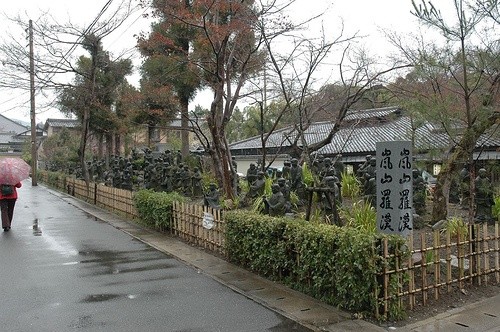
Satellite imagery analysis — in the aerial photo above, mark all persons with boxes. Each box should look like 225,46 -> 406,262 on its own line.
262,180 -> 291,217
191,166 -> 203,199
305,154 -> 340,225
460,168 -> 496,226
105,147 -> 154,190
195,150 -> 203,173
333,155 -> 344,182
356,155 -> 377,209
0,182 -> 22,232
46,159 -> 106,182
177,149 -> 182,165
413,169 -> 426,214
144,150 -> 175,193
203,183 -> 222,214
231,156 -> 237,172
272,158 -> 305,200
244,163 -> 272,204
172,163 -> 193,197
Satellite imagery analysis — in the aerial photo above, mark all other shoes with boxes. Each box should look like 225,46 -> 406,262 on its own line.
4,227 -> 10,231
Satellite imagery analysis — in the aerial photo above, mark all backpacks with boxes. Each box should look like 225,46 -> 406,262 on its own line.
2,184 -> 15,196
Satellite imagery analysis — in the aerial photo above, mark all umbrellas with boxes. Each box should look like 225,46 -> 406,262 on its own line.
0,157 -> 32,185
